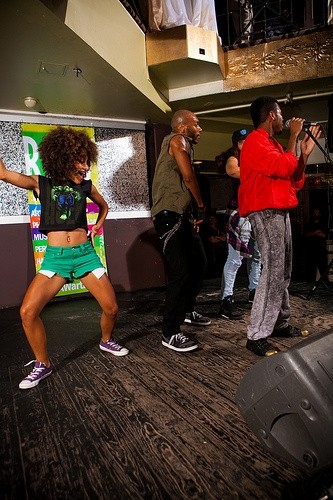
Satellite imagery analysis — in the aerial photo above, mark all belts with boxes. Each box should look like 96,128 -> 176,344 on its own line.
159,211 -> 173,215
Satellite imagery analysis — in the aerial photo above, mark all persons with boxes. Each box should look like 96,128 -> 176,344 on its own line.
214,128 -> 261,321
0,127 -> 129,390
151,111 -> 211,351
238,96 -> 321,356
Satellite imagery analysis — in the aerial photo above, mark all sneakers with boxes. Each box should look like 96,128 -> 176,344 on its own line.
218,295 -> 242,320
184,311 -> 211,325
246,338 -> 278,357
248,289 -> 256,303
99,337 -> 129,356
272,325 -> 309,337
19,358 -> 52,389
161,332 -> 198,352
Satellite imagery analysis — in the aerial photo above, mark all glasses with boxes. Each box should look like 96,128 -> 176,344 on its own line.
236,129 -> 247,138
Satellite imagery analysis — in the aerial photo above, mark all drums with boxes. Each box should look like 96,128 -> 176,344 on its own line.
295,175 -> 333,237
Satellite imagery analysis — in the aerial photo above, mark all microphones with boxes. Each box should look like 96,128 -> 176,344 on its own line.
285,119 -> 318,127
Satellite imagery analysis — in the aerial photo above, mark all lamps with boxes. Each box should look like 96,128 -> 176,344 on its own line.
24,97 -> 38,108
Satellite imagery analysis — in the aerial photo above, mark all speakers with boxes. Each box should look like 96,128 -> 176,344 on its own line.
236,326 -> 333,474
